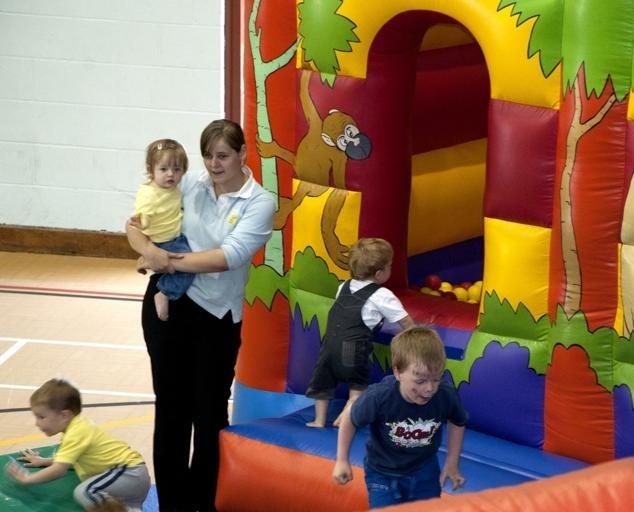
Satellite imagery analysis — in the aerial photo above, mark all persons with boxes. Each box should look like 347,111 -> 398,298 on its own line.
305,235 -> 418,428
333,323 -> 467,509
124,119 -> 277,512
131,137 -> 196,320
5,378 -> 151,511
